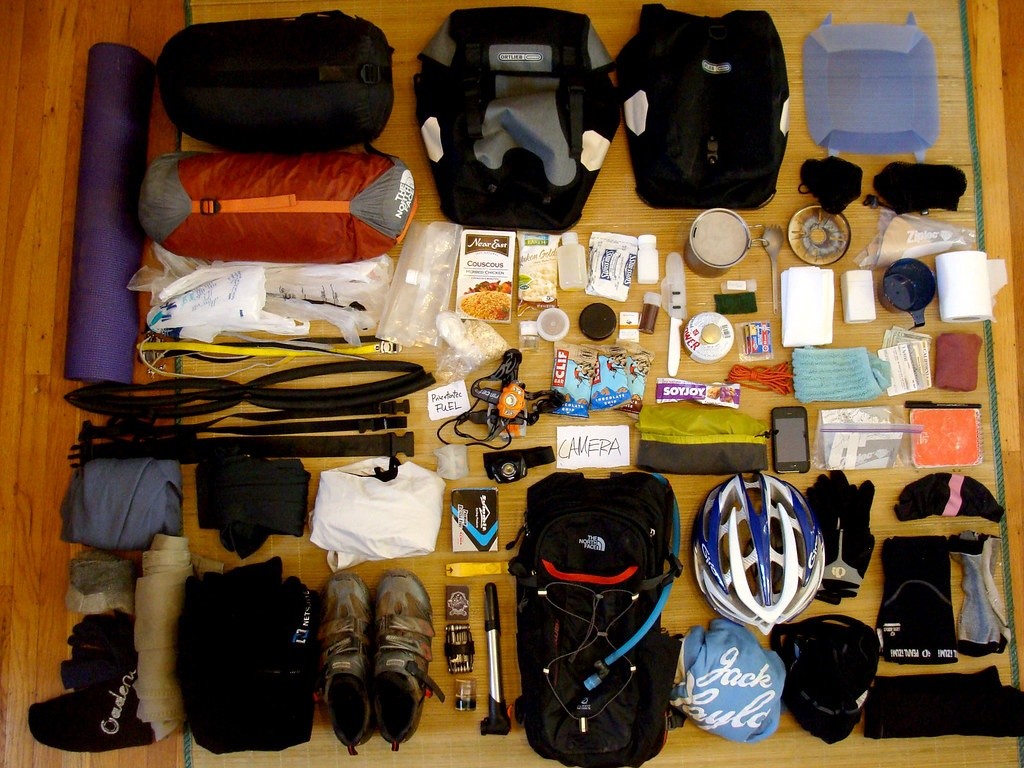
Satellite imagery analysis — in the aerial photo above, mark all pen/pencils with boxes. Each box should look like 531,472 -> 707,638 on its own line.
905,400 -> 981,412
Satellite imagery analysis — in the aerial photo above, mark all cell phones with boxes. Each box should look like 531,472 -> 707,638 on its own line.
771,406 -> 811,474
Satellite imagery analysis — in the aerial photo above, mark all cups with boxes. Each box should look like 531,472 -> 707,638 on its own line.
683,208 -> 770,278
876,258 -> 936,332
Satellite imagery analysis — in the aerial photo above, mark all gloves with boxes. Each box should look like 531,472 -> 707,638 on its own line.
805,467 -> 875,605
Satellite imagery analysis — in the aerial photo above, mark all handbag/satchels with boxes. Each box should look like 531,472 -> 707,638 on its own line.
619,3 -> 792,211
154,10 -> 395,151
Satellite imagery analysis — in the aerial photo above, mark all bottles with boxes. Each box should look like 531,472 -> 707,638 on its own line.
558,231 -> 587,291
637,235 -> 660,284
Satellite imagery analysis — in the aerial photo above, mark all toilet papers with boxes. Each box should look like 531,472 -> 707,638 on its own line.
840,268 -> 877,324
936,250 -> 1008,323
780,264 -> 836,347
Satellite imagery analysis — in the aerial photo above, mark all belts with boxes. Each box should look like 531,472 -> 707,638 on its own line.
135,334 -> 400,379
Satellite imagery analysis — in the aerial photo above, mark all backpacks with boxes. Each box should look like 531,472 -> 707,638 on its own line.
410,5 -> 617,235
507,470 -> 682,768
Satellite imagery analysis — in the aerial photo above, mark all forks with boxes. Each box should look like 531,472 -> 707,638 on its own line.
762,224 -> 784,314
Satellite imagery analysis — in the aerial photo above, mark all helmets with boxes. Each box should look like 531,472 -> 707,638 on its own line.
691,471 -> 826,633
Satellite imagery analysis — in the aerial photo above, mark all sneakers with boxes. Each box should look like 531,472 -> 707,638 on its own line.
320,574 -> 374,756
371,567 -> 445,751
